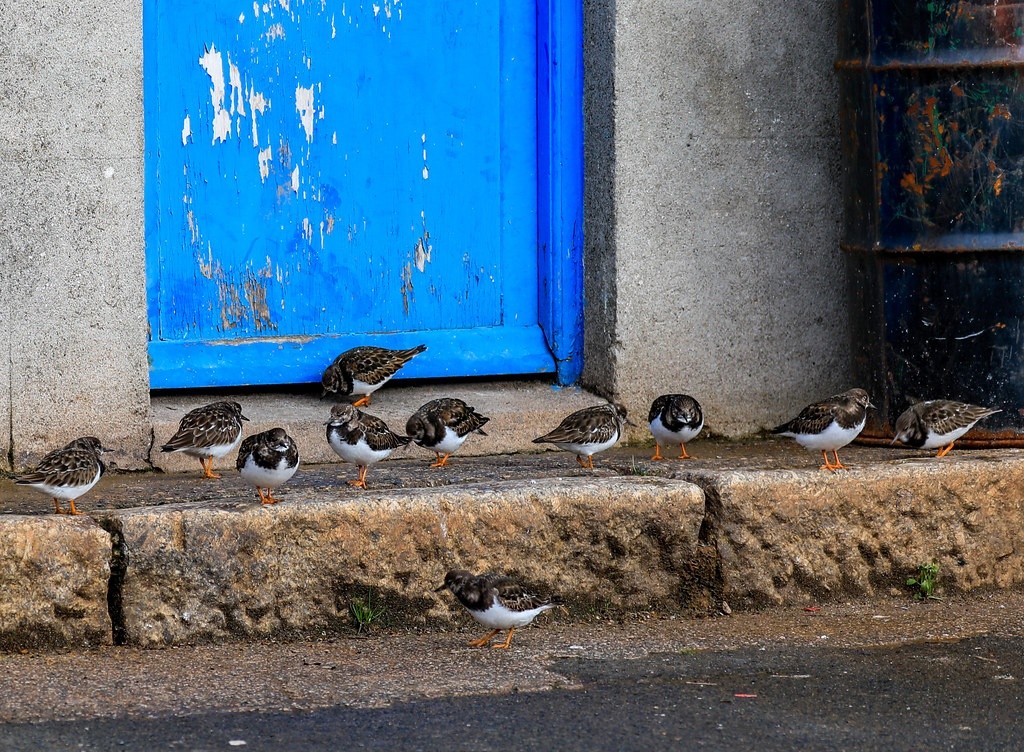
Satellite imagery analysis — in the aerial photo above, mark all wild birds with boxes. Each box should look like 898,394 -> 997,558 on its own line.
434,568 -> 568,651
233,428 -> 300,505
158,400 -> 250,478
323,402 -> 413,490
319,344 -> 430,406
532,403 -> 638,472
649,393 -> 705,462
770,388 -> 879,474
404,397 -> 490,468
14,437 -> 113,516
892,399 -> 1005,459
